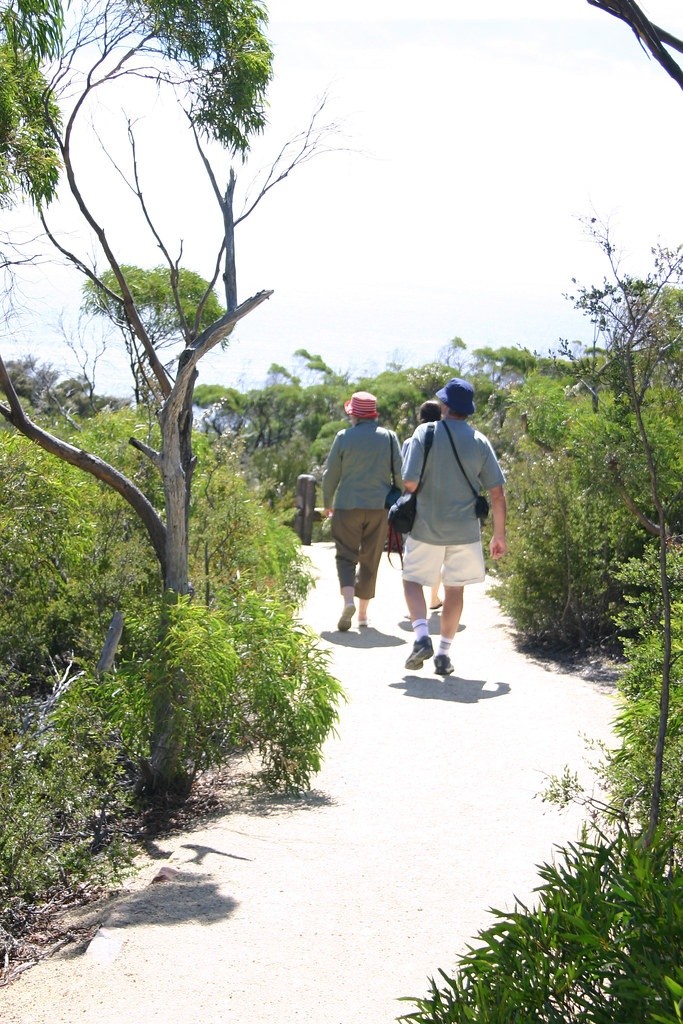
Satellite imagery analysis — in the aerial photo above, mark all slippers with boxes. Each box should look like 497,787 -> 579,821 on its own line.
430,600 -> 442,610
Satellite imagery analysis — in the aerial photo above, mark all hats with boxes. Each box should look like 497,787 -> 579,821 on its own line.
436,377 -> 476,414
344,391 -> 379,417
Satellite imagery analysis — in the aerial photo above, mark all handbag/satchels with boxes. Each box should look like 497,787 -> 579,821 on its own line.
388,494 -> 416,534
475,497 -> 488,518
384,485 -> 401,511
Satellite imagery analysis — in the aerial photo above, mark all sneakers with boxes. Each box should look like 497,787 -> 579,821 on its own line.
434,655 -> 454,675
405,636 -> 434,671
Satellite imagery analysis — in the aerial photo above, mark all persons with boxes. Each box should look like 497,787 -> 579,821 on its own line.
320,378 -> 509,676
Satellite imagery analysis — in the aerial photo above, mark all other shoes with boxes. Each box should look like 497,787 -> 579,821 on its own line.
359,622 -> 366,627
338,602 -> 356,631
